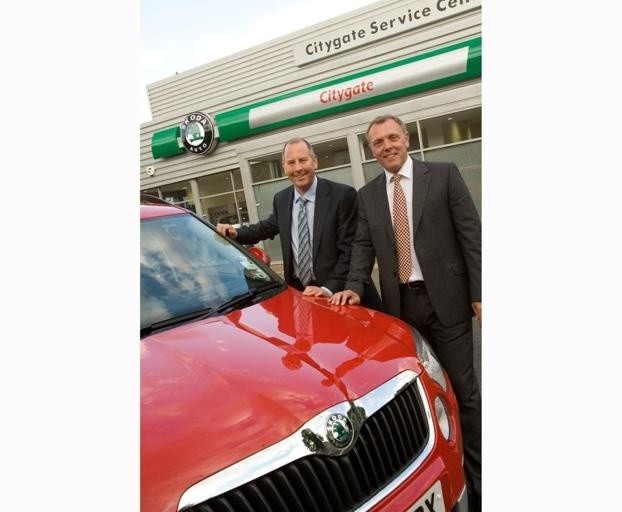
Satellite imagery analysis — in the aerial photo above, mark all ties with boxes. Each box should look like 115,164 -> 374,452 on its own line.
297,197 -> 312,287
392,175 -> 411,284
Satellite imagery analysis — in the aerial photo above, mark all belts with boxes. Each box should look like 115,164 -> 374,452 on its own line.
400,281 -> 424,290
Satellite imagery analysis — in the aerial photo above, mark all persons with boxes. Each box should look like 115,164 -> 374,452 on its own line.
328,114 -> 481,511
215,138 -> 382,311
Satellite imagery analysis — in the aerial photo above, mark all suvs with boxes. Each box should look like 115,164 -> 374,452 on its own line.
141,194 -> 468,511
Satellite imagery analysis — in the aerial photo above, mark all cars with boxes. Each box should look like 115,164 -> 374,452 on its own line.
215,209 -> 273,227
